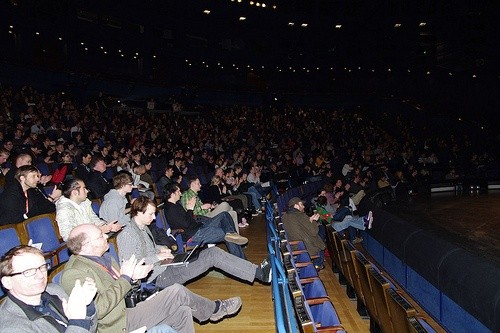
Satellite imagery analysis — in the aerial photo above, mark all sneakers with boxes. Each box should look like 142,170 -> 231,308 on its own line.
224,232 -> 248,245
210,297 -> 241,323
259,253 -> 271,284
367,211 -> 374,230
237,199 -> 268,228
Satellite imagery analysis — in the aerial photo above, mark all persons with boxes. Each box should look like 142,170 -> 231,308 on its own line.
60,224 -> 243,333
0,82 -> 500,260
116,195 -> 272,288
0,245 -> 179,333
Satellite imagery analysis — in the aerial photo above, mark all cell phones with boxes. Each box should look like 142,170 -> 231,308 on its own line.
56,181 -> 64,190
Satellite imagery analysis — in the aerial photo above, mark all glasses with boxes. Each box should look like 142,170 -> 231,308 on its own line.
127,182 -> 135,186
4,264 -> 50,279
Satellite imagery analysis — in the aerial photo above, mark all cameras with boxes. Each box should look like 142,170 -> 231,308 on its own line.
124,287 -> 147,308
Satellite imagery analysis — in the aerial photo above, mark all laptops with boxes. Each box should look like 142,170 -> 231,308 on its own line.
160,240 -> 204,266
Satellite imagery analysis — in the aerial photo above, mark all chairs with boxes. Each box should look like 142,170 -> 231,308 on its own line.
264,180 -> 493,333
0,183 -> 201,310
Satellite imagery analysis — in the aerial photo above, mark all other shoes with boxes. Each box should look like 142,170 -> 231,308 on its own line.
315,264 -> 328,273
